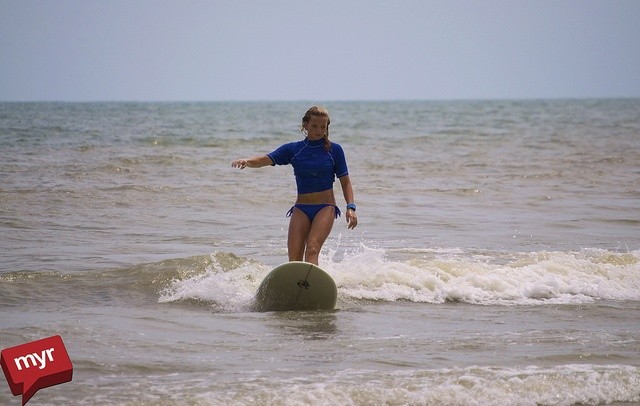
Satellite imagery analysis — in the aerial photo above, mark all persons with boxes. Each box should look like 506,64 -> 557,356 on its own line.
231,107 -> 357,266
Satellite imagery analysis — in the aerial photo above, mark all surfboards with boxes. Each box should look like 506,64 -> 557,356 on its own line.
254,261 -> 337,309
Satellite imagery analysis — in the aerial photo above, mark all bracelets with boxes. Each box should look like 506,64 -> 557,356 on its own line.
347,204 -> 356,210
